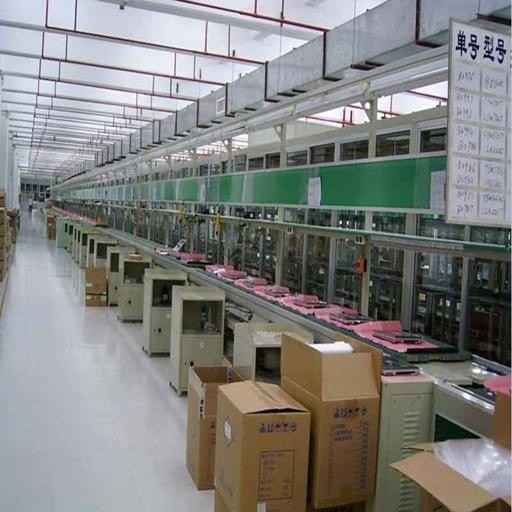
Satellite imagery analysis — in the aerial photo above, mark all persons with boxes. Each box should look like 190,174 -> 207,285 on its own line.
43,193 -> 54,226
39,193 -> 44,213
28,194 -> 35,215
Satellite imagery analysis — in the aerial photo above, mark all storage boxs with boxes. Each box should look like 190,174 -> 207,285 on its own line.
0,187 -> 19,282
181,338 -> 383,508
390,382 -> 510,511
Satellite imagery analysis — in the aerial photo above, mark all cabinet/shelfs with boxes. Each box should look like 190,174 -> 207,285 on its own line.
64,217 -> 225,396
